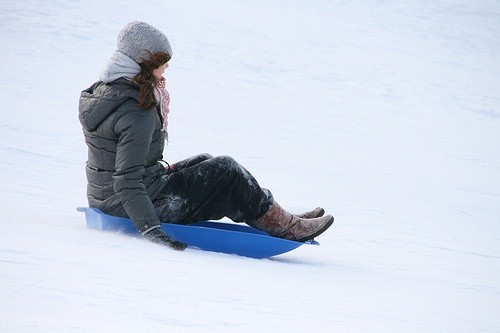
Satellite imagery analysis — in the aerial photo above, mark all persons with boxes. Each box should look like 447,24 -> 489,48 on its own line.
77,21 -> 334,251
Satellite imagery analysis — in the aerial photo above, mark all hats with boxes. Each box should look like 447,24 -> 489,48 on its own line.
116,20 -> 173,63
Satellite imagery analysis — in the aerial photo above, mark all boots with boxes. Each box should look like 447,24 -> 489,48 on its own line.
246,188 -> 334,242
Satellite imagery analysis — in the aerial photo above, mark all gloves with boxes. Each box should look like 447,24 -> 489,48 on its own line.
152,235 -> 187,251
